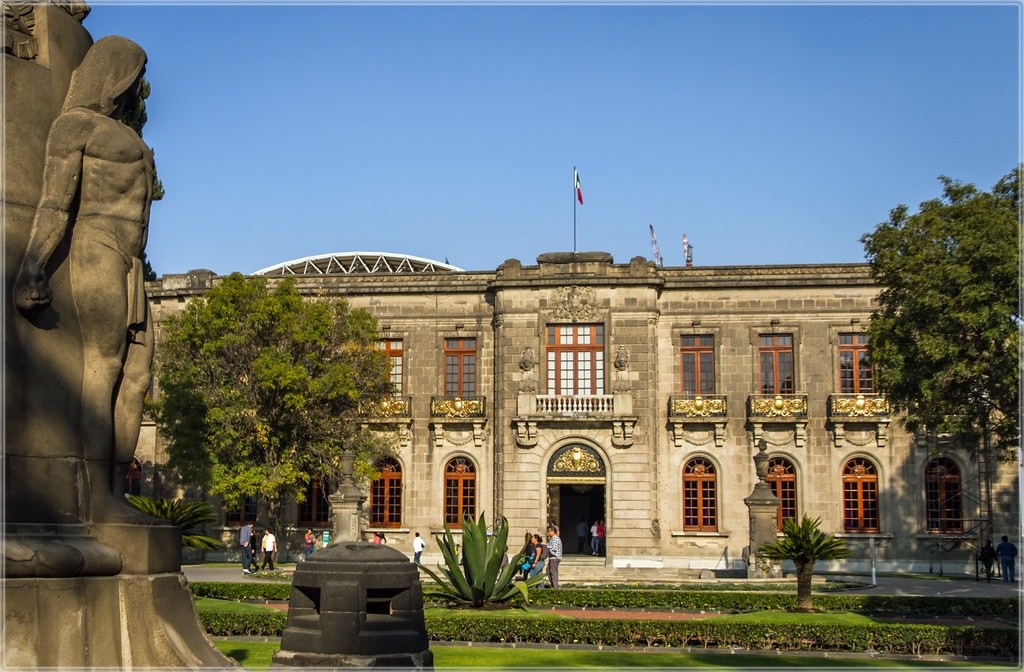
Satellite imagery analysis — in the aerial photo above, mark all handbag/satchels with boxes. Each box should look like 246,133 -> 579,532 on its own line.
421,544 -> 424,548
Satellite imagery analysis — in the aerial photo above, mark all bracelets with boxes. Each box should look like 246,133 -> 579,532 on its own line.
533,562 -> 536,564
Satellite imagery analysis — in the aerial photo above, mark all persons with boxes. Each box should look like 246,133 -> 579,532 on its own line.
413,532 -> 425,564
576,518 -> 604,556
13,35 -> 172,526
373,531 -> 386,545
978,535 -> 1018,583
304,528 -> 316,556
530,526 -> 562,588
239,521 -> 278,575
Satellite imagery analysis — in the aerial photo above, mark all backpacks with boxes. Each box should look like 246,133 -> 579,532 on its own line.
535,544 -> 548,559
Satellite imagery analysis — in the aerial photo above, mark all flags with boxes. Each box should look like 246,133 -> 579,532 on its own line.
575,169 -> 584,205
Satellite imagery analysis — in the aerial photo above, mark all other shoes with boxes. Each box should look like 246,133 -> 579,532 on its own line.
243,569 -> 251,575
593,552 -> 598,556
